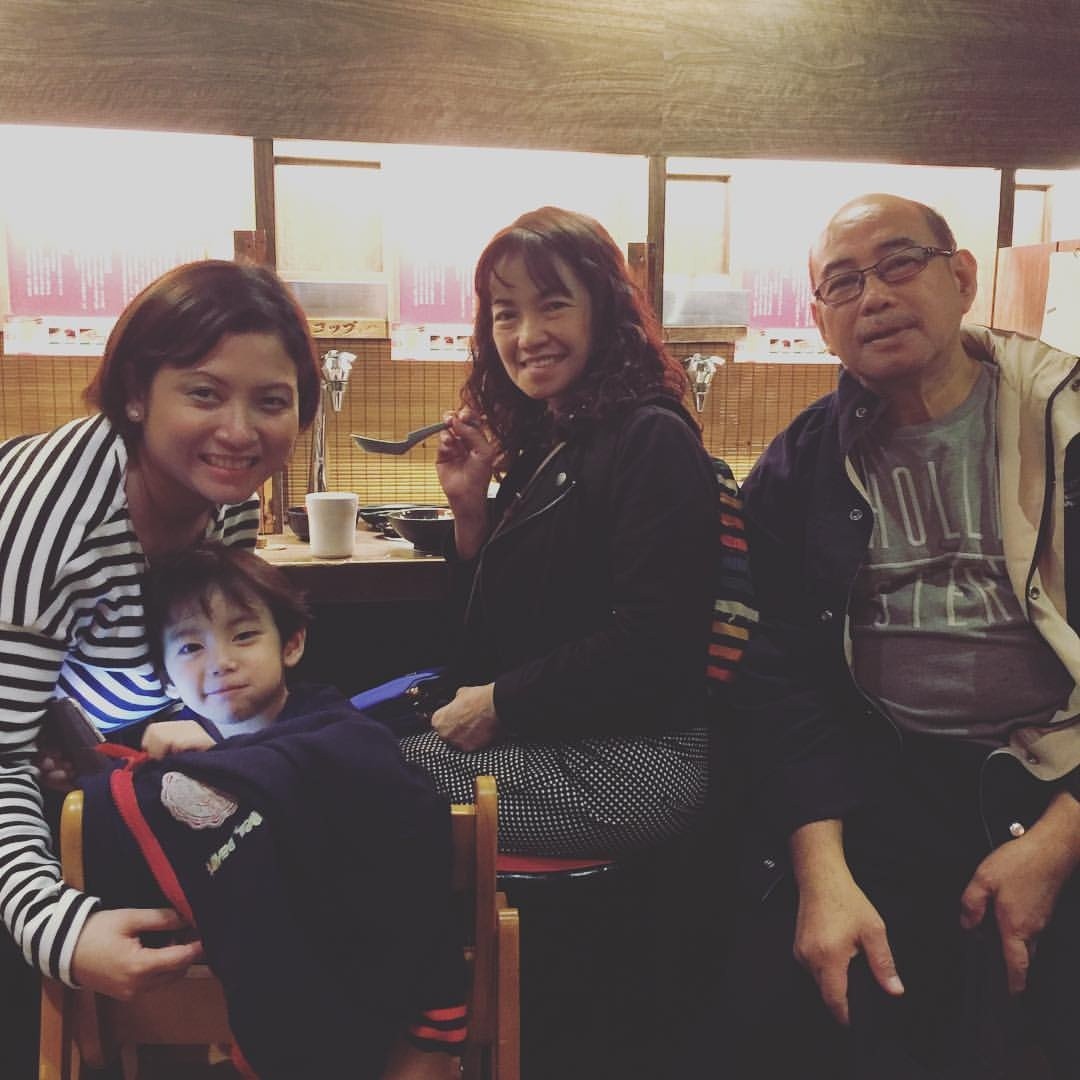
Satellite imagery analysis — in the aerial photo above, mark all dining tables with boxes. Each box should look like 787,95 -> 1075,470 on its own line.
253,529 -> 457,609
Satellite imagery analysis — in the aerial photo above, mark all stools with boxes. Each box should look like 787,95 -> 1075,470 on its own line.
487,853 -> 661,1080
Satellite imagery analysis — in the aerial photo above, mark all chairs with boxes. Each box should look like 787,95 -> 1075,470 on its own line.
38,773 -> 521,1080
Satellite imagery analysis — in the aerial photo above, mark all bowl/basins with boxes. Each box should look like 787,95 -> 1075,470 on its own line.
388,507 -> 455,555
287,492 -> 419,556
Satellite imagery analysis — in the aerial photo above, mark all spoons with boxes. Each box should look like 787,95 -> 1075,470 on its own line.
350,422 -> 452,456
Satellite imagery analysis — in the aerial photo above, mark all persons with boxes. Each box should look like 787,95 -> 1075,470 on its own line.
38,542 -> 367,845
722,191 -> 1080,1080
374,205 -> 739,897
0,248 -> 328,1080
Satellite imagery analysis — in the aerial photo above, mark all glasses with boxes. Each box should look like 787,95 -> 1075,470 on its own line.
813,246 -> 953,306
